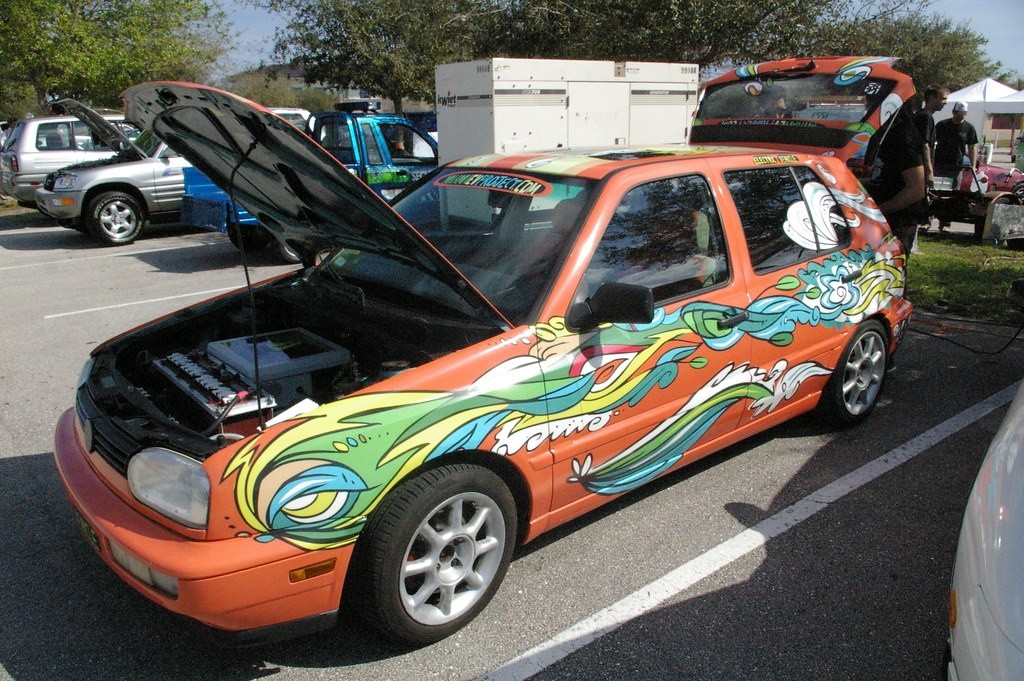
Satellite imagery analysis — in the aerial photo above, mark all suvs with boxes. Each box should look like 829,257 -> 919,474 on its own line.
33,106 -> 326,245
0,113 -> 144,207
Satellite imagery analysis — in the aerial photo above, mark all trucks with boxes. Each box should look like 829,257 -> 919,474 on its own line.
178,101 -> 442,265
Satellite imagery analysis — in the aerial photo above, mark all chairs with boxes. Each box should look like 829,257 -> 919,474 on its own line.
615,208 -> 716,290
512,198 -> 589,303
382,134 -> 414,159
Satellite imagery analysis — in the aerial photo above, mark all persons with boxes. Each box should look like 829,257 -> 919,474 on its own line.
863,83 -> 978,260
391,125 -> 411,158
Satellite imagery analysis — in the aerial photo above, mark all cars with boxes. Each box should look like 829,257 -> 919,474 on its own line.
49,57 -> 918,650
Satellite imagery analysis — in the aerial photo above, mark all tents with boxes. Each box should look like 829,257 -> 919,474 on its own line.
923,77 -> 1024,152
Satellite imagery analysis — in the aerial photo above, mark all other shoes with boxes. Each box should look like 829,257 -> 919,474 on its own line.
918,224 -> 930,234
940,226 -> 950,235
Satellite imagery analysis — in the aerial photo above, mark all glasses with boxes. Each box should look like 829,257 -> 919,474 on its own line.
757,104 -> 786,118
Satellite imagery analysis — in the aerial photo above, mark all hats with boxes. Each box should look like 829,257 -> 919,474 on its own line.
954,101 -> 968,115
